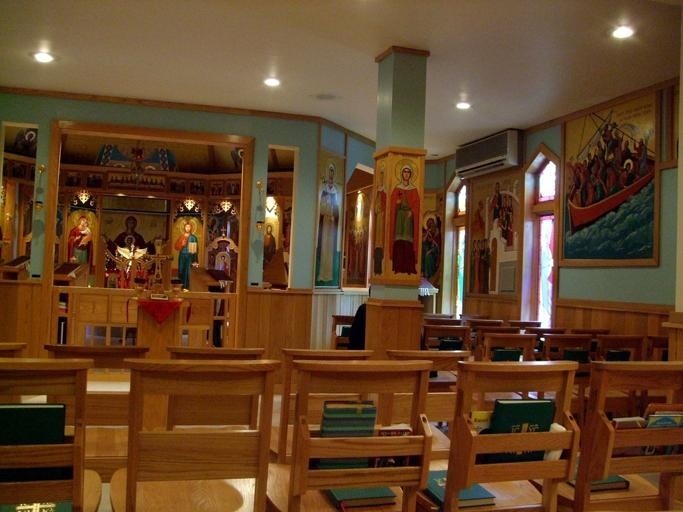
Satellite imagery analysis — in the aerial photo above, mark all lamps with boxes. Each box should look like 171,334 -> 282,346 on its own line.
77,187 -> 90,205
220,199 -> 233,213
182,194 -> 196,211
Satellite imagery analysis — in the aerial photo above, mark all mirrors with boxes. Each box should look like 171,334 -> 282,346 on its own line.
261,144 -> 300,290
0,120 -> 41,285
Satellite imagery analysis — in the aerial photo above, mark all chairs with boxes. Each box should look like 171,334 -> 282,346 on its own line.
417,361 -> 581,511
1,341 -> 376,471
110,358 -> 281,512
531,360 -> 682,511
329,313 -> 668,464
266,360 -> 435,512
1,358 -> 102,512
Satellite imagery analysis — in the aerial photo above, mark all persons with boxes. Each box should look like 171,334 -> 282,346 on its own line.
369,167 -> 386,276
310,165 -> 339,283
77,222 -> 92,247
173,221 -> 198,289
422,216 -> 442,278
264,225 -> 275,264
389,164 -> 418,273
216,255 -> 229,289
103,216 -> 161,290
566,121 -> 651,209
206,219 -> 218,241
470,182 -> 514,296
67,216 -> 94,275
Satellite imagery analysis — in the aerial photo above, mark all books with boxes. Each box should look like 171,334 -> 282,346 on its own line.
432,327 -> 669,377
0,401 -> 69,482
320,485 -> 398,511
422,466 -> 495,510
561,454 -> 630,494
608,410 -> 682,457
483,399 -> 556,462
372,422 -> 412,468
316,398 -> 377,471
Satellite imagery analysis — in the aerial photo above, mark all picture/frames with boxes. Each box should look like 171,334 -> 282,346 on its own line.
558,83 -> 662,268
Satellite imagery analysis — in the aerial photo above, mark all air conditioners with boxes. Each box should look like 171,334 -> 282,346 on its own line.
454,129 -> 524,180
